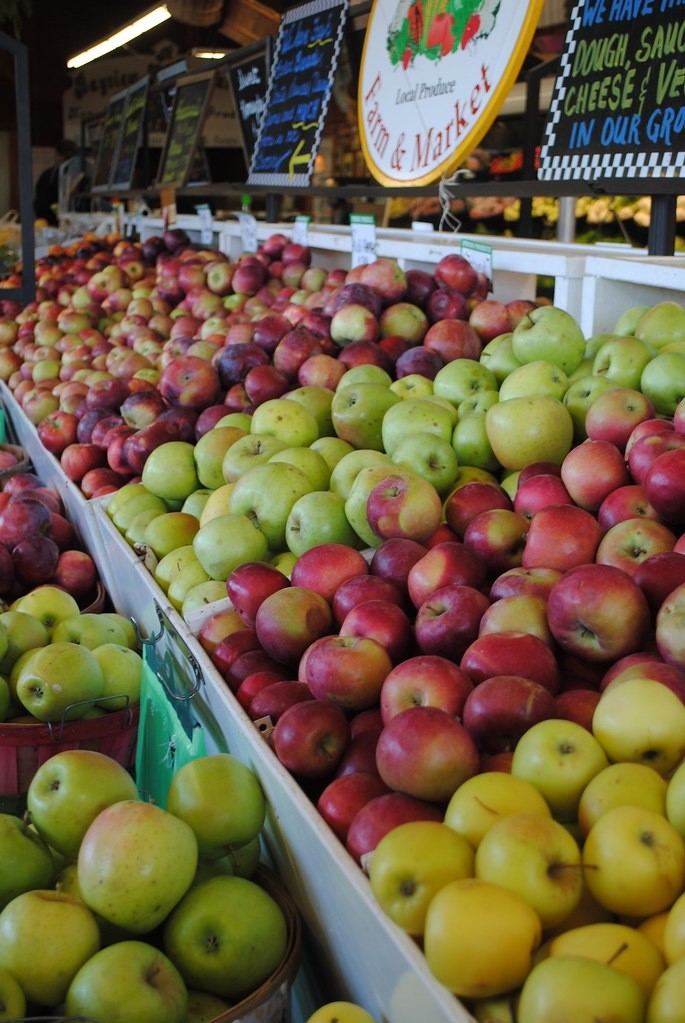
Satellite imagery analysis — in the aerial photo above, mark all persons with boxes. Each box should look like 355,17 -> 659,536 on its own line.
33,139 -> 97,228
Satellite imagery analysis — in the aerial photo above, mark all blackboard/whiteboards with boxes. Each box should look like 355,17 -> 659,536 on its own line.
153,70 -> 220,186
225,51 -> 273,173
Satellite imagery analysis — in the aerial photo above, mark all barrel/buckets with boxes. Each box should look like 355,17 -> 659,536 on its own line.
1,442 -> 297,1023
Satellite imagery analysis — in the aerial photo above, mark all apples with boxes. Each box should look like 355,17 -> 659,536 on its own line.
0,196 -> 685,1023
